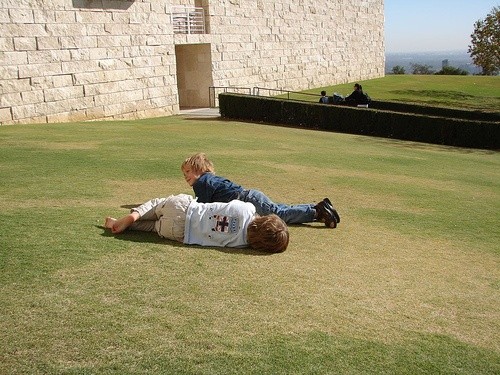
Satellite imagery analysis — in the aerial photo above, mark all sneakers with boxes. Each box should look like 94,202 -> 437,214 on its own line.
312,197 -> 340,229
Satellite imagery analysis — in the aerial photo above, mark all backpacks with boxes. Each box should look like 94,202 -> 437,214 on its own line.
356,89 -> 372,104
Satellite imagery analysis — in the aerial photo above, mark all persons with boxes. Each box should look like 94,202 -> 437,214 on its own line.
105,193 -> 290,254
181,152 -> 340,229
319,90 -> 330,104
345,83 -> 367,107
